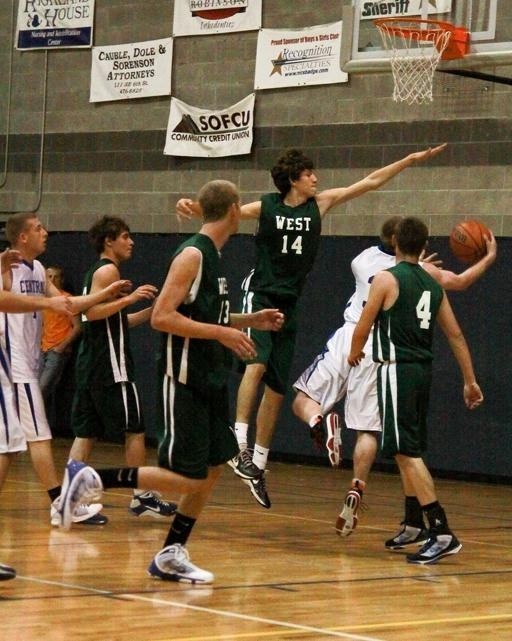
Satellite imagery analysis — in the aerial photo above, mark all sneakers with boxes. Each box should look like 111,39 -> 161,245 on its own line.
242,471 -> 270,509
0,561 -> 14,581
128,494 -> 177,518
385,524 -> 432,550
78,512 -> 108,525
60,459 -> 94,530
335,488 -> 361,538
321,413 -> 343,467
406,531 -> 462,565
227,451 -> 261,480
150,547 -> 213,585
50,498 -> 102,526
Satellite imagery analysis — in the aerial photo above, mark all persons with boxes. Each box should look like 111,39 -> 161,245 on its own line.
348,217 -> 485,565
176,141 -> 450,510
292,220 -> 497,538
1,246 -> 76,580
37,262 -> 82,395
58,180 -> 285,584
62,217 -> 179,519
1,212 -> 133,527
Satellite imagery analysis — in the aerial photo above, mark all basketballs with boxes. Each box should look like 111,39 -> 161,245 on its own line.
450,220 -> 491,263
